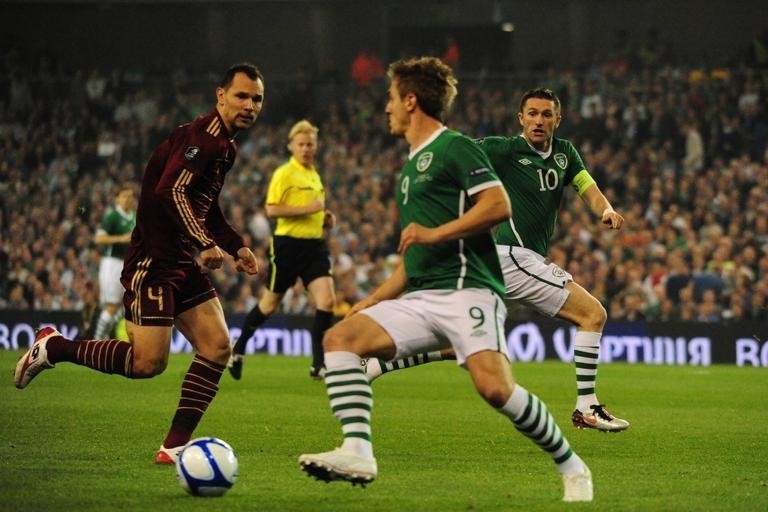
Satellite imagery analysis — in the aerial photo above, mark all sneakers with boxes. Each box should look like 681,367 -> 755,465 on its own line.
227,344 -> 244,380
560,462 -> 594,502
12,326 -> 62,390
155,444 -> 185,464
572,405 -> 630,433
309,364 -> 326,377
298,447 -> 377,488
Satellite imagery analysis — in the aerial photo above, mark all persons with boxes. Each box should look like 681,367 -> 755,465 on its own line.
2,49 -> 768,324
296,58 -> 591,502
221,119 -> 335,380
12,70 -> 263,458
469,89 -> 632,434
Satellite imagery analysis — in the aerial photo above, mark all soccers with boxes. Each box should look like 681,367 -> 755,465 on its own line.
177,437 -> 237,497
